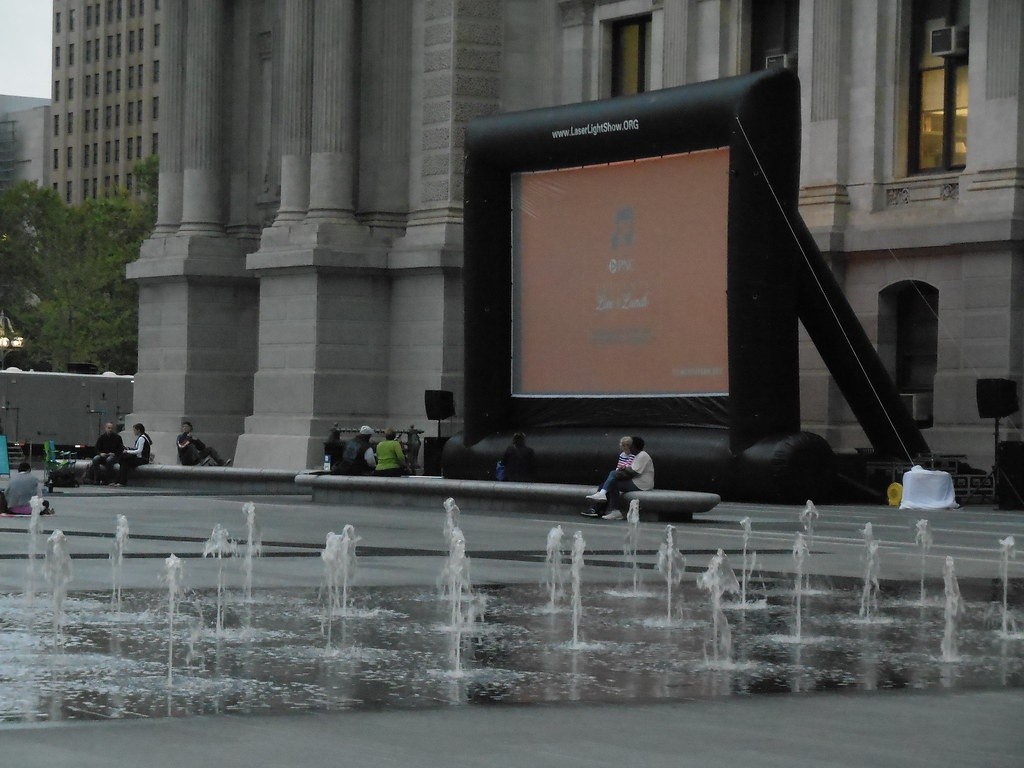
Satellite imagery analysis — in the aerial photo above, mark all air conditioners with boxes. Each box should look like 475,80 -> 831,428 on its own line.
928,25 -> 964,57
765,53 -> 797,70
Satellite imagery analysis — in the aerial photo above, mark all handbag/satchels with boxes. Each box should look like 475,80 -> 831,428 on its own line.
495,462 -> 504,481
615,468 -> 633,480
402,464 -> 411,475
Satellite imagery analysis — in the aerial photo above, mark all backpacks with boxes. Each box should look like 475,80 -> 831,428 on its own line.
342,439 -> 364,472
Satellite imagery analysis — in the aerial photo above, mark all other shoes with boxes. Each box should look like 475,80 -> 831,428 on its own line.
224,459 -> 231,465
200,458 -> 210,465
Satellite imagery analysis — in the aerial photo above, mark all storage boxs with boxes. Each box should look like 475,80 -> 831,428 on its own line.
892,458 -> 996,497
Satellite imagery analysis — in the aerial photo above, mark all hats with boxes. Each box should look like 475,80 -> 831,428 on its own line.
360,426 -> 375,434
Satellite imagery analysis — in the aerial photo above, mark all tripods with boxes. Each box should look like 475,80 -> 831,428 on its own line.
960,419 -> 1024,510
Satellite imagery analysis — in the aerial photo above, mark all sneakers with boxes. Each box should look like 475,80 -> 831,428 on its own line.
601,511 -> 623,520
585,492 -> 607,503
580,509 -> 598,516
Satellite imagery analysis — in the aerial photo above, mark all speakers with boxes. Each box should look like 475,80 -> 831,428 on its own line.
976,379 -> 1018,418
424,390 -> 455,420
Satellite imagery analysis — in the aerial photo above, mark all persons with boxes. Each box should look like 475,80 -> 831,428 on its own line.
580,436 -> 655,520
374,426 -> 412,477
342,426 -> 376,476
3,463 -> 49,513
92,423 -> 124,486
176,421 -> 231,466
108,423 -> 153,487
501,432 -> 536,483
